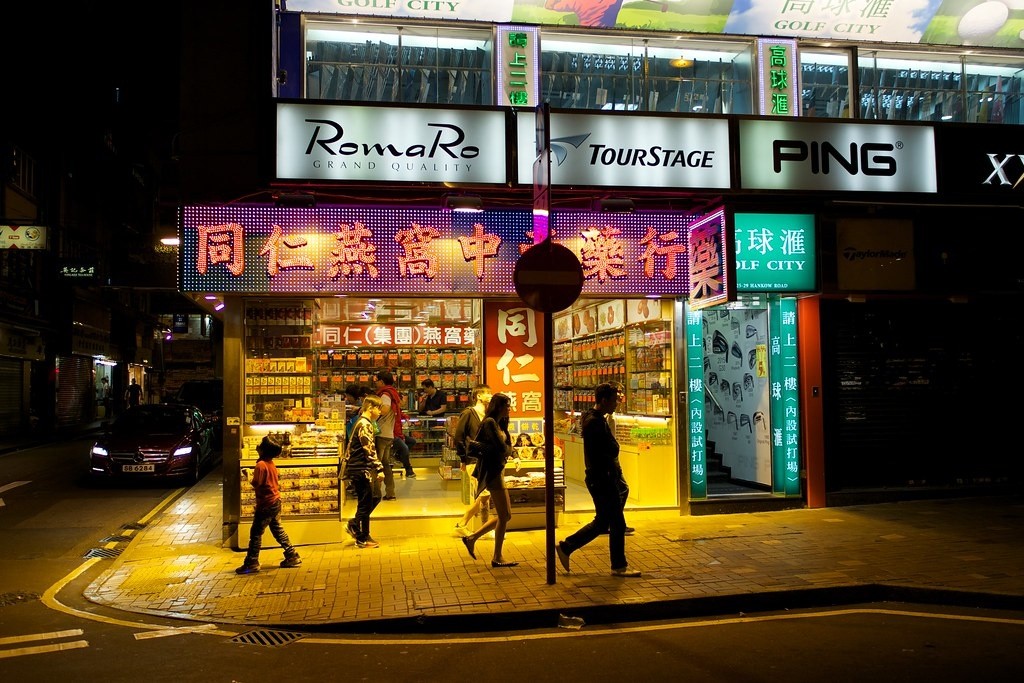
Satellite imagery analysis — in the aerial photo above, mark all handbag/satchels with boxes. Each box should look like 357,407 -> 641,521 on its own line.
338,457 -> 350,481
372,420 -> 381,435
466,440 -> 491,459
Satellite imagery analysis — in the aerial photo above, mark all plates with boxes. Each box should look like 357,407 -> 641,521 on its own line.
510,433 -> 563,461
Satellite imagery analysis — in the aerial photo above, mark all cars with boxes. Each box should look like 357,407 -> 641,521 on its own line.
87,404 -> 220,486
176,379 -> 223,451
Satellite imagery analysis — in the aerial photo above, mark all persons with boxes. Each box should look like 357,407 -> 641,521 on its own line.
124,379 -> 144,408
101,378 -> 112,418
344,394 -> 385,549
453,384 -> 506,540
603,380 -> 635,535
463,392 -> 519,568
235,435 -> 303,575
417,379 -> 447,451
344,371 -> 416,500
555,383 -> 641,577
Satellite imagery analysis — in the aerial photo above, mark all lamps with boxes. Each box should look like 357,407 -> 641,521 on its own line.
277,189 -> 317,207
599,197 -> 637,216
441,190 -> 484,212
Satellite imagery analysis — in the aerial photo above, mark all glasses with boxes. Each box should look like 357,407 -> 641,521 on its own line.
375,379 -> 381,384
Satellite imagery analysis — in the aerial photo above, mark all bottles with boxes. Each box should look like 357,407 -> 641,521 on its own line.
574,331 -> 627,414
268,430 -> 291,458
317,349 -> 479,412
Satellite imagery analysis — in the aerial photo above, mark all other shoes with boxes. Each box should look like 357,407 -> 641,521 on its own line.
383,496 -> 396,500
491,558 -> 519,568
478,530 -> 506,539
463,536 -> 476,560
598,529 -> 610,536
405,472 -> 416,478
353,492 -> 358,498
625,527 -> 634,534
454,522 -> 472,539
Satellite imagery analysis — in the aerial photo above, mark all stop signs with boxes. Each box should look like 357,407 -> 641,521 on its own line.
513,243 -> 584,312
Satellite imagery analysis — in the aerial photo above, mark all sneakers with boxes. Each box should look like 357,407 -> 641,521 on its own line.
235,562 -> 260,574
611,564 -> 642,576
554,544 -> 571,573
344,518 -> 358,540
280,555 -> 301,567
354,535 -> 379,549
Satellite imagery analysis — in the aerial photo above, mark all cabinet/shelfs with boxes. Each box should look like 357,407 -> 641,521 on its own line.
402,417 -> 446,457
470,459 -> 567,532
243,297 -> 321,425
553,319 -> 672,417
436,429 -> 462,492
318,345 -> 477,413
